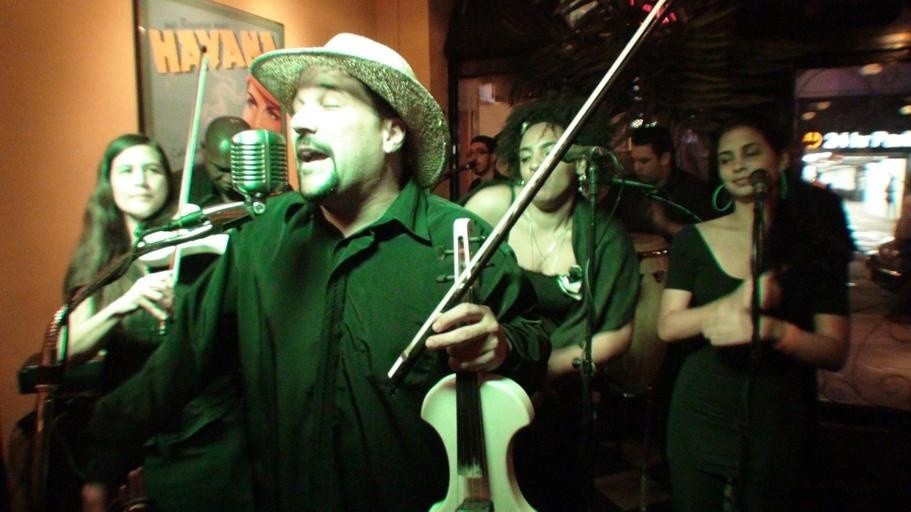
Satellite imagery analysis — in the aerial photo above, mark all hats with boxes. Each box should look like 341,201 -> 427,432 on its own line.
248,32 -> 451,189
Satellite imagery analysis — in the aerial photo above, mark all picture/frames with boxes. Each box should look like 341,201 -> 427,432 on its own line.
132,1 -> 288,175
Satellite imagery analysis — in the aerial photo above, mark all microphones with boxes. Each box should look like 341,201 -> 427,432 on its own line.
229,128 -> 290,201
749,168 -> 774,198
561,142 -> 603,163
454,160 -> 477,175
599,173 -> 656,189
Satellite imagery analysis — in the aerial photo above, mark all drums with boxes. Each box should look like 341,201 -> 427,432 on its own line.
599,233 -> 671,394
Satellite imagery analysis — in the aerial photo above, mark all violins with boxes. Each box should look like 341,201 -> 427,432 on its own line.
137,191 -> 289,267
421,219 -> 536,511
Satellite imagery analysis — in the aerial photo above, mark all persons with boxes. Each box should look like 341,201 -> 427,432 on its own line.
242,75 -> 281,133
467,135 -> 509,190
600,127 -> 707,235
80,63 -> 552,512
57,134 -> 175,364
656,115 -> 850,512
173,116 -> 252,207
463,97 -> 640,376
